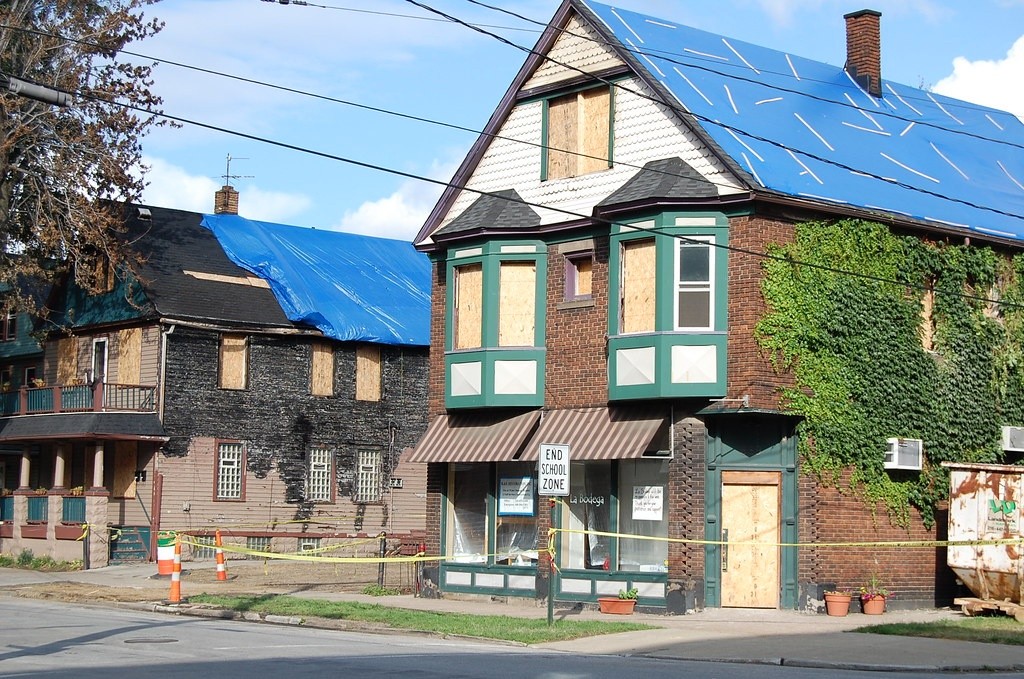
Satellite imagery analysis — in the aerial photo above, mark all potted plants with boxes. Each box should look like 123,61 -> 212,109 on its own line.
597,588 -> 639,614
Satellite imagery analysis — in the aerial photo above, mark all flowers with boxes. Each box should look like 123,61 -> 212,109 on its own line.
860,575 -> 895,609
823,589 -> 854,595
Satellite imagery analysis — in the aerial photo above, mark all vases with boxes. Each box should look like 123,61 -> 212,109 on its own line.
825,593 -> 851,616
862,595 -> 886,615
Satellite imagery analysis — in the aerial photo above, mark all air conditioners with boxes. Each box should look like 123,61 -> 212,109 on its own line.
996,426 -> 1024,452
882,438 -> 923,470
